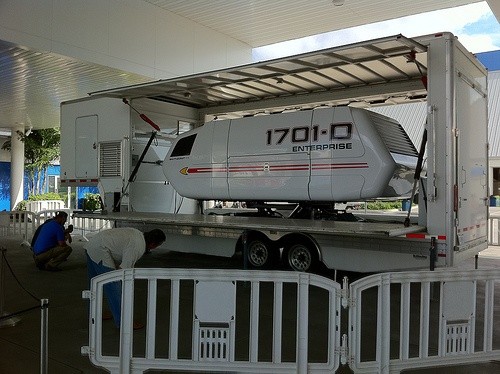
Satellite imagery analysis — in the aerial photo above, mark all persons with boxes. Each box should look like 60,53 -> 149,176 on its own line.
30,214 -> 72,259
82,227 -> 166,330
33,211 -> 73,272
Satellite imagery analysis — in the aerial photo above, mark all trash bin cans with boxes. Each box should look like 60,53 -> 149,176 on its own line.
401,200 -> 411,211
491,195 -> 500,207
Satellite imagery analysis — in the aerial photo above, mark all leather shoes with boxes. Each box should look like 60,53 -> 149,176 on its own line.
133,322 -> 143,328
102,313 -> 112,320
